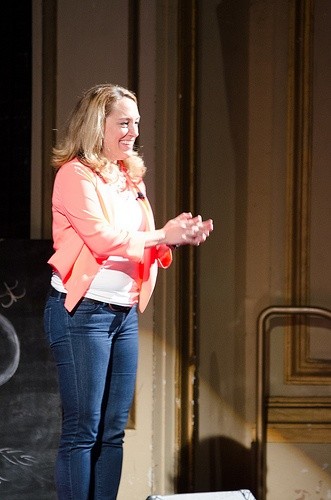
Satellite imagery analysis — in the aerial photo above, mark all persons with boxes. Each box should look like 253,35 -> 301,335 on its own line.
41,82 -> 216,500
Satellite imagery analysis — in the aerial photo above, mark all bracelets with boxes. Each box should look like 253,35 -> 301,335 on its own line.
172,244 -> 180,250
